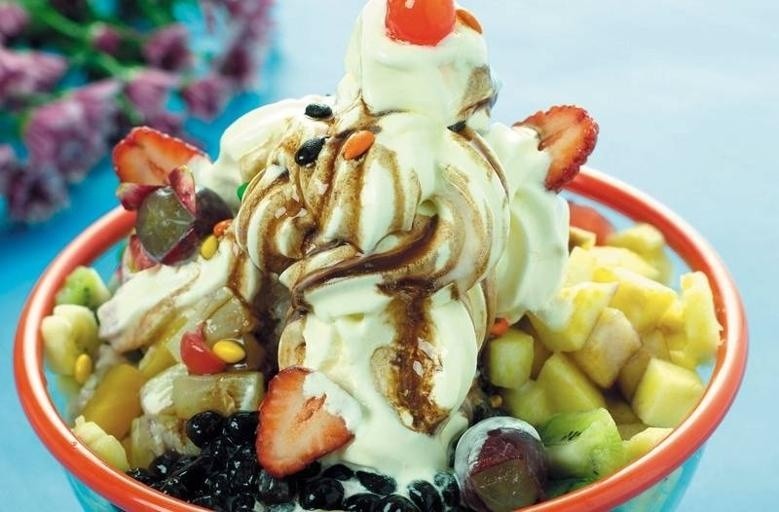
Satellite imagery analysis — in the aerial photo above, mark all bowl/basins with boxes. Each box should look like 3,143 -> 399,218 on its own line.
14,165 -> 750,512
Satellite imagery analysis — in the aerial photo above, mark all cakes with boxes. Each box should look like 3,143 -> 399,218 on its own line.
42,0 -> 719,510
